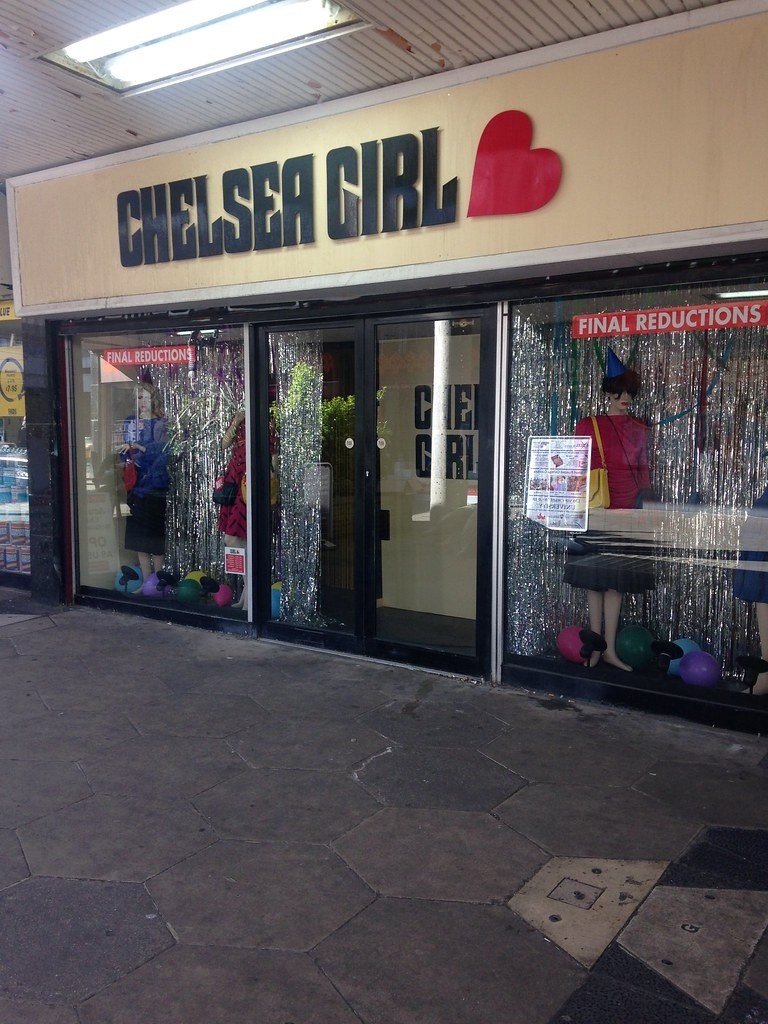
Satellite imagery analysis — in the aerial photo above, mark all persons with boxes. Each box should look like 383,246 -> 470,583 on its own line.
118,381 -> 281,610
735,490 -> 768,696
559,346 -> 650,673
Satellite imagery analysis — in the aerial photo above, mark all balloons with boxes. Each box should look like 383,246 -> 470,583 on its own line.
560,620 -> 718,687
115,565 -> 230,608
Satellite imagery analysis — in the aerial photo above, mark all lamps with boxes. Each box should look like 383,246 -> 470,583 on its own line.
118,565 -> 140,593
578,629 -> 607,670
61,0 -> 343,84
651,639 -> 685,679
155,570 -> 173,601
198,576 -> 220,605
735,654 -> 768,700
